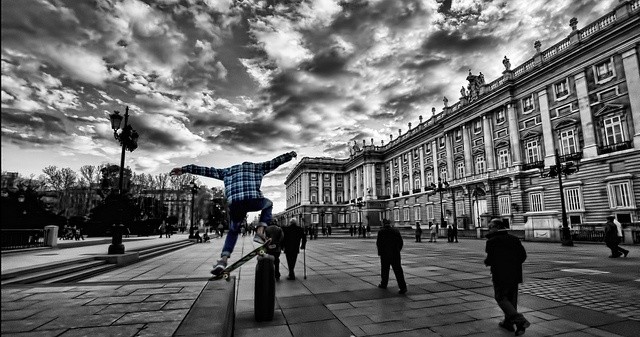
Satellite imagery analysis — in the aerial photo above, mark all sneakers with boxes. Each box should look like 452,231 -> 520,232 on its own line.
499,321 -> 515,332
210,260 -> 227,275
514,320 -> 530,336
379,283 -> 386,288
253,231 -> 269,244
399,288 -> 407,294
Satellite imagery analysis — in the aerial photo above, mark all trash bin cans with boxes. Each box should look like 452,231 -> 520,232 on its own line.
44,225 -> 59,246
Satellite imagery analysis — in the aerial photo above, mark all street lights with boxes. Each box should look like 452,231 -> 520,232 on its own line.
299,213 -> 302,227
431,177 -> 449,227
109,106 -> 140,254
548,149 -> 576,246
356,201 -> 366,235
189,179 -> 199,239
320,210 -> 325,228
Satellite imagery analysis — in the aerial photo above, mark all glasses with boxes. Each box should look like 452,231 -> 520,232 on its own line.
489,226 -> 497,229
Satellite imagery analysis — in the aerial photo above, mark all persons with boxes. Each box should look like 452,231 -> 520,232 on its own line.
447,222 -> 459,243
165,222 -> 170,237
59,223 -> 85,240
429,223 -> 436,242
484,218 -> 530,336
376,217 -> 407,294
307,223 -> 318,239
415,226 -> 422,241
281,218 -> 307,279
349,223 -> 371,238
604,216 -> 630,257
610,215 -> 623,256
195,230 -> 202,242
169,150 -> 297,275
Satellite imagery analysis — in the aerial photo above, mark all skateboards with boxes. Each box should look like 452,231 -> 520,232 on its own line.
208,238 -> 273,281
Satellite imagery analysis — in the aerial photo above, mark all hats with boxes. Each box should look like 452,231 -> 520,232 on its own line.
488,221 -> 504,228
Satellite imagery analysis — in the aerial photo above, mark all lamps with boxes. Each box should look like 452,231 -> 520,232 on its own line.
445,209 -> 451,215
510,203 -> 519,211
